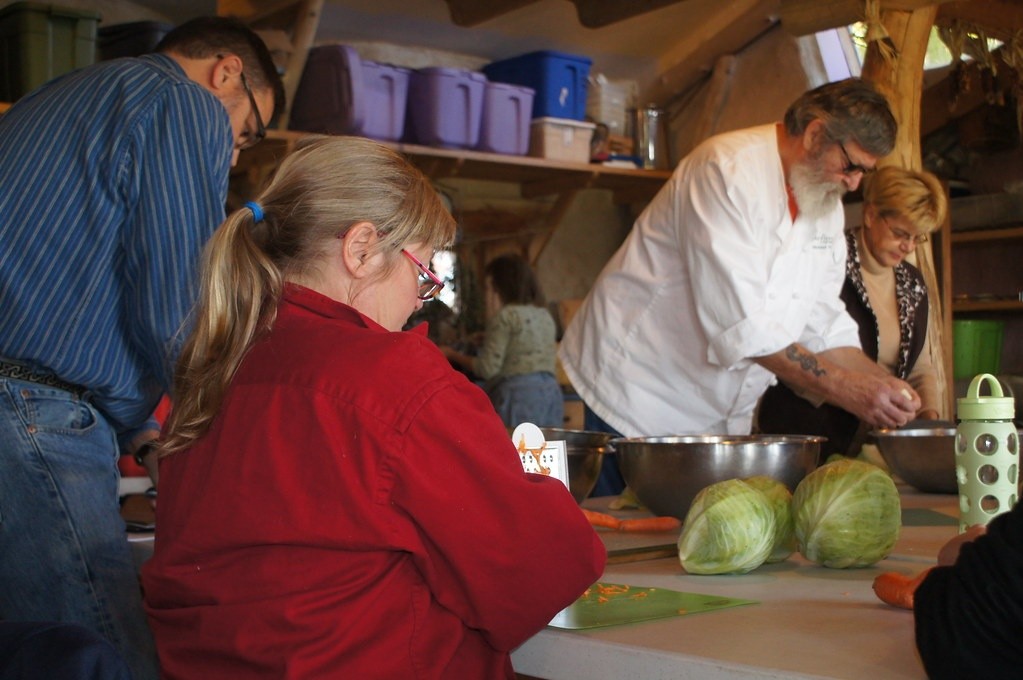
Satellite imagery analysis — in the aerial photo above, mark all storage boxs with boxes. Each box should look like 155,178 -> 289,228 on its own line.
289,50 -> 597,166
952,320 -> 1005,382
0,0 -> 174,102
951,192 -> 1023,230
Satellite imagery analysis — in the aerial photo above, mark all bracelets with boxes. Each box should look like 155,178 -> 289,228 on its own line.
135,438 -> 158,465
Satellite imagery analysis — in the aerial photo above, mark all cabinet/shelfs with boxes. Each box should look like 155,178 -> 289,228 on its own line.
941,181 -> 1023,426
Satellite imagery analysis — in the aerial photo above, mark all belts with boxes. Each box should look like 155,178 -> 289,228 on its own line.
0,361 -> 97,408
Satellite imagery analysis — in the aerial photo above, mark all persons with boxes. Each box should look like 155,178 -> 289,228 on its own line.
558,76 -> 923,498
913,491 -> 1023,680
758,168 -> 944,464
0,16 -> 286,680
142,136 -> 605,680
440,254 -> 564,427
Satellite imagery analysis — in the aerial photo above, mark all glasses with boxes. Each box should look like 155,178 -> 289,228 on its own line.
839,141 -> 877,175
338,230 -> 445,300
883,216 -> 928,245
216,54 -> 265,152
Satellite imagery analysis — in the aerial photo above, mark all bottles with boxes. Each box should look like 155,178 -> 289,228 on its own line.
625,96 -> 669,170
953,372 -> 1019,535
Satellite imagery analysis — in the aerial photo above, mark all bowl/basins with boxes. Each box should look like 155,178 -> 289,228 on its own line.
866,423 -> 961,496
507,425 -> 617,504
607,432 -> 829,520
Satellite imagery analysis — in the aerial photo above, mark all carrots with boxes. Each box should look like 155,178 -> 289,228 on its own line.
871,566 -> 932,610
581,508 -> 683,531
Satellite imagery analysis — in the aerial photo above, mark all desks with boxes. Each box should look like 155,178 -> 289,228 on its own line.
126,473 -> 1023,680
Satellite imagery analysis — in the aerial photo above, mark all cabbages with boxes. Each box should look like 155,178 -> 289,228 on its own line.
678,459 -> 902,576
